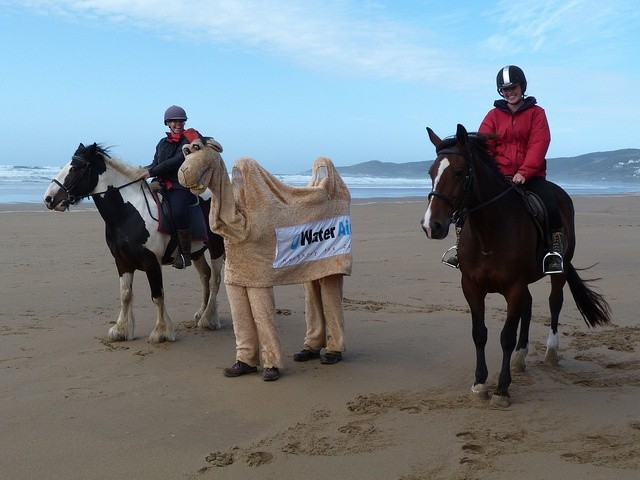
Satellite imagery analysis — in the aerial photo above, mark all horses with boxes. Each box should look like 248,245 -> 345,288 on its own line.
43,142 -> 226,343
421,124 -> 612,408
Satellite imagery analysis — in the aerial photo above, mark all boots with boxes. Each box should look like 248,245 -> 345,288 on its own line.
172,225 -> 191,269
548,232 -> 568,271
447,227 -> 462,266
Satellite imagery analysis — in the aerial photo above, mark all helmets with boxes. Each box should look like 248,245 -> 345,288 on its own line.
497,66 -> 527,95
164,105 -> 187,125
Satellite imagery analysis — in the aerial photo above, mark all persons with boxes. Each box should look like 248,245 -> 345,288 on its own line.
446,66 -> 566,274
140,105 -> 195,268
178,137 -> 356,382
